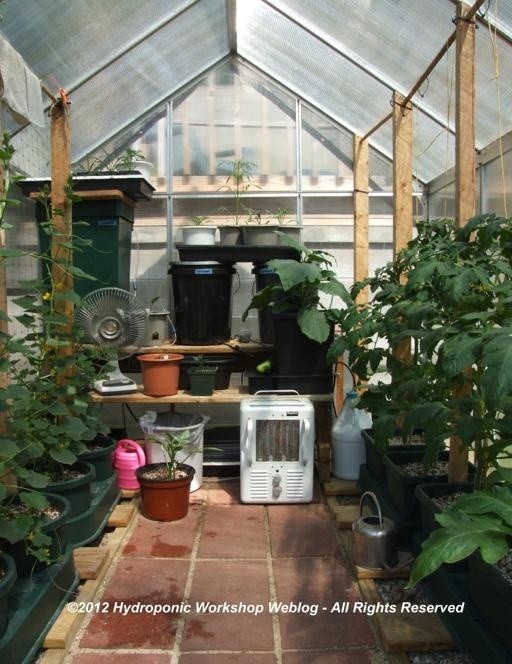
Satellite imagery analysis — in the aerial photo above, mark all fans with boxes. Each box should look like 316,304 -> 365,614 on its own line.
73,289 -> 147,395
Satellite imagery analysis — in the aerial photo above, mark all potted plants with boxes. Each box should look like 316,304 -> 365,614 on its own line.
0,129 -> 127,636
245,230 -> 352,376
113,146 -> 156,181
137,344 -> 219,397
134,430 -> 227,521
178,158 -> 302,249
324,193 -> 510,663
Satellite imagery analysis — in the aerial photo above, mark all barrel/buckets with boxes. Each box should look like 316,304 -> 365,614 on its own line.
250,265 -> 320,345
166,260 -> 235,345
331,391 -> 374,479
271,309 -> 335,375
109,439 -> 144,493
